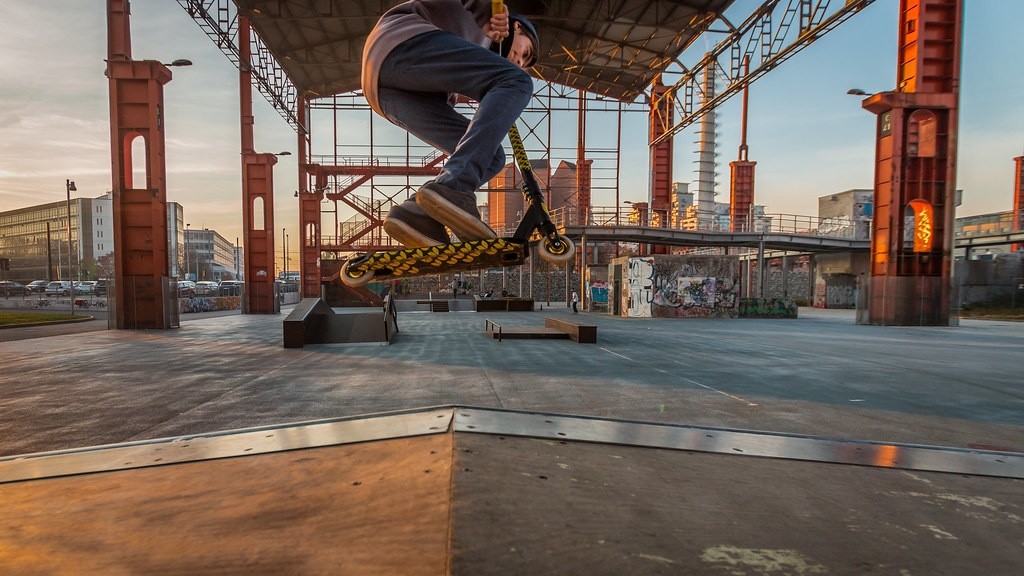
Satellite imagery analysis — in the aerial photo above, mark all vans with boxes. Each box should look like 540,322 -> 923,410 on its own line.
219,281 -> 244,296
196,281 -> 218,295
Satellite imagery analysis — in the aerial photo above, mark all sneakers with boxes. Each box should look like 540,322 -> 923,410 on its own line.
383,206 -> 453,248
415,180 -> 498,242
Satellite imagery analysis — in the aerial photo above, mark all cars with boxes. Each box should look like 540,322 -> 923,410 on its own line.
46,281 -> 82,296
27,280 -> 49,294
76,281 -> 96,296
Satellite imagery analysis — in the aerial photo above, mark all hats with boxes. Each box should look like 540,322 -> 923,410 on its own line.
511,14 -> 540,66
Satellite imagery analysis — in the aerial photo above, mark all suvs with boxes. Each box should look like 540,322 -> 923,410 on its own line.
178,281 -> 197,299
95,278 -> 115,297
0,281 -> 32,297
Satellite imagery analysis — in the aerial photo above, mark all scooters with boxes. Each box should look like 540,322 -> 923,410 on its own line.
341,0 -> 577,289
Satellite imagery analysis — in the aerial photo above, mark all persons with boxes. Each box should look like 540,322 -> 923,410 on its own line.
450,278 -> 517,298
570,288 -> 578,313
361,0 -> 540,249
986,248 -> 992,254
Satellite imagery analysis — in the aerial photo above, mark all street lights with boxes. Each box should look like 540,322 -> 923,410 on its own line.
64,177 -> 85,319
